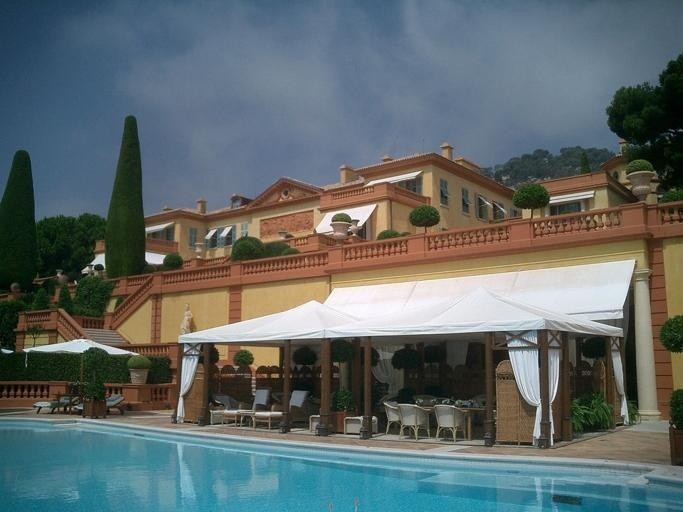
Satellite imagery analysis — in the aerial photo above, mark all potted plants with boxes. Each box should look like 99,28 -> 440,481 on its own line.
624,158 -> 655,202
570,392 -> 613,440
126,353 -> 148,384
331,388 -> 361,434
81,383 -> 107,420
666,387 -> 683,466
329,212 -> 354,241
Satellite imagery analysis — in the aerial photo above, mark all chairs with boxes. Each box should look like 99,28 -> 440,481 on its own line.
31,393 -> 127,420
206,387 -> 313,430
380,395 -> 485,441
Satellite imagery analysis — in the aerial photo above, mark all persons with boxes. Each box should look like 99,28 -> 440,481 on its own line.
179,302 -> 194,336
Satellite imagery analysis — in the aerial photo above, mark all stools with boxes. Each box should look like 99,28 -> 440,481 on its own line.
341,415 -> 377,436
308,414 -> 320,432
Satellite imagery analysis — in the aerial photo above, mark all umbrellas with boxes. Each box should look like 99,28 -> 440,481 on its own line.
21,338 -> 141,394
